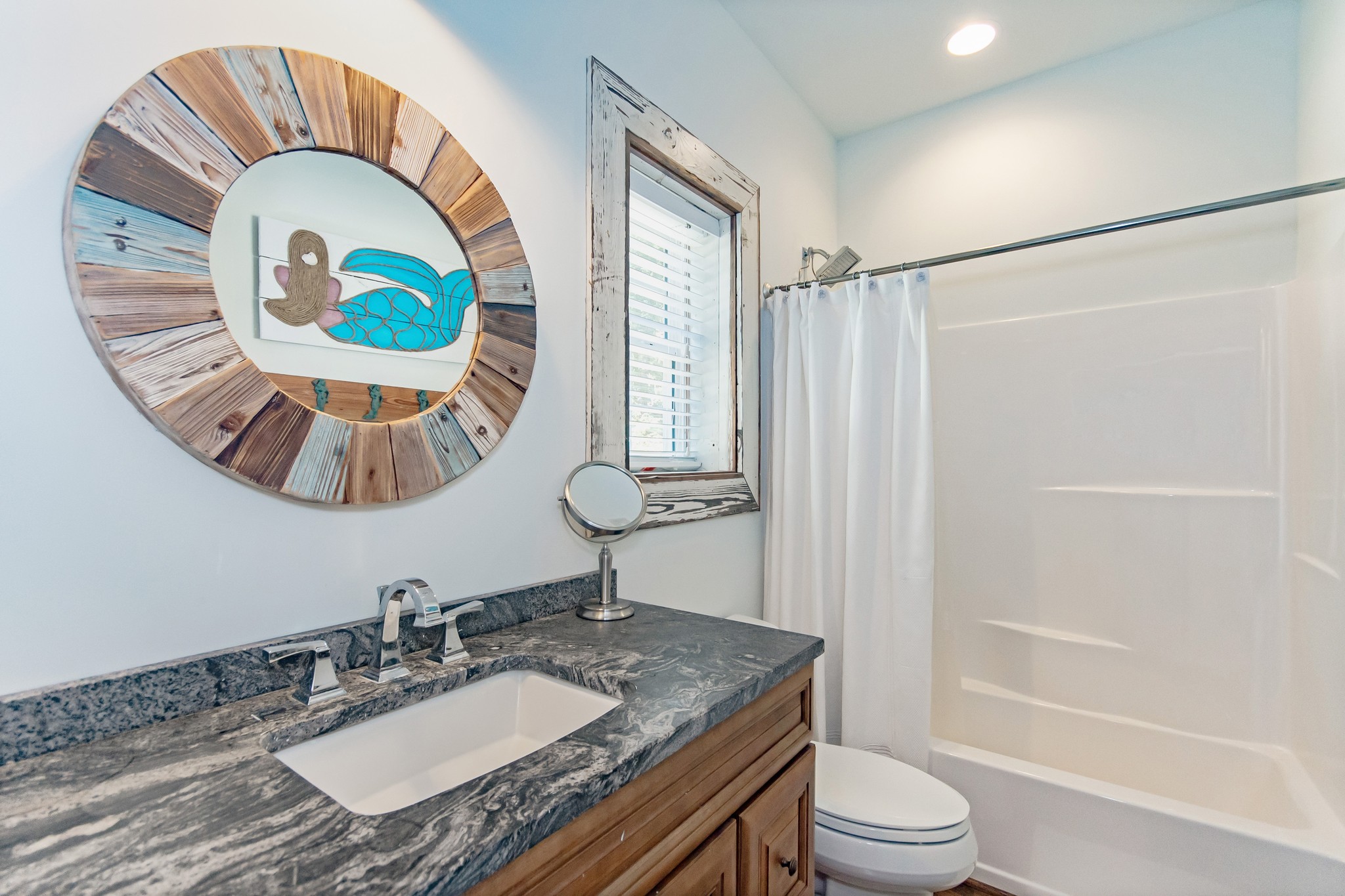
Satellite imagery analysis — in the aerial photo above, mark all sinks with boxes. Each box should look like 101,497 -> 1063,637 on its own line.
257,657 -> 636,821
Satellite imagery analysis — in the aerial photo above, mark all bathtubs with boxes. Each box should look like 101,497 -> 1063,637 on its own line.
933,676 -> 1345,895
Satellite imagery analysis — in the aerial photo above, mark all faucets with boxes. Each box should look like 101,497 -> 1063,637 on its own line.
360,577 -> 444,684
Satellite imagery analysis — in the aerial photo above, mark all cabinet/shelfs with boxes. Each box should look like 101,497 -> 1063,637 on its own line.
474,662 -> 818,896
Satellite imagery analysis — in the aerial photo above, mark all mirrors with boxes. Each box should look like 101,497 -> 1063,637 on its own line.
557,460 -> 651,623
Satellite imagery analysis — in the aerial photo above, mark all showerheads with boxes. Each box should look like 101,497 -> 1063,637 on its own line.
816,245 -> 862,289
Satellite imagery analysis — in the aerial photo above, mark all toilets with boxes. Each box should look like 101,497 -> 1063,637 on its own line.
810,738 -> 981,896
